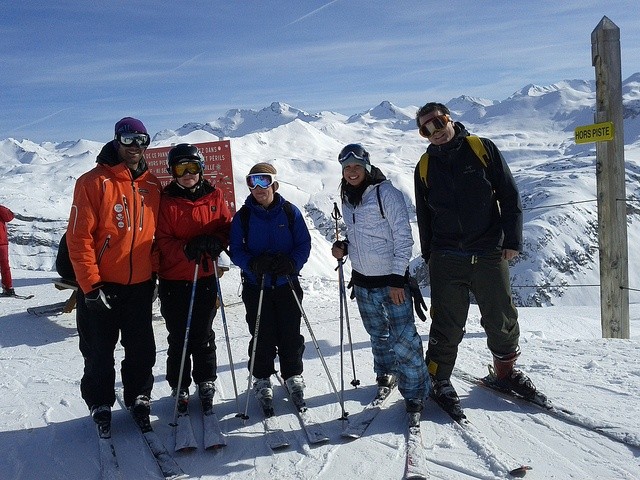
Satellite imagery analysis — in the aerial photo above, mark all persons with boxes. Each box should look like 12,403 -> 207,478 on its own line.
228,162 -> 310,400
57,231 -> 80,287
0,205 -> 14,291
332,143 -> 431,413
66,117 -> 163,423
155,142 -> 231,404
414,103 -> 537,405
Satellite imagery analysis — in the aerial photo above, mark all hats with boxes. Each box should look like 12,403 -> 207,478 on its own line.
342,144 -> 372,174
114,117 -> 147,136
248,162 -> 279,192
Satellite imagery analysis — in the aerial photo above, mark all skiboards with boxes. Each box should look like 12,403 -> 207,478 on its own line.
429,367 -> 640,475
27,302 -> 76,316
252,372 -> 330,450
174,413 -> 228,454
96,387 -> 189,480
338,376 -> 429,480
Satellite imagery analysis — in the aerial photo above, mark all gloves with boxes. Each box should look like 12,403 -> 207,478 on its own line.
254,256 -> 277,275
85,290 -> 117,310
279,256 -> 296,276
204,237 -> 223,256
151,274 -> 160,303
410,278 -> 428,321
347,277 -> 357,300
183,234 -> 207,260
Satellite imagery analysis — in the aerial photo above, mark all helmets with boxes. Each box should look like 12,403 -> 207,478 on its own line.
169,143 -> 205,170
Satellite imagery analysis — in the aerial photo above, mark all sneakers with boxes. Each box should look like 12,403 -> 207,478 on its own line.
253,376 -> 273,398
403,395 -> 425,412
133,394 -> 150,416
379,371 -> 398,385
494,370 -> 536,397
285,375 -> 306,391
173,388 -> 189,401
199,380 -> 215,398
2,285 -> 15,295
427,377 -> 460,406
90,403 -> 112,422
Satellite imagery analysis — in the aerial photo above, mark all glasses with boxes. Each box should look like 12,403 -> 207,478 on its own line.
419,114 -> 453,138
113,134 -> 151,148
168,160 -> 204,178
246,173 -> 278,190
338,143 -> 370,162
